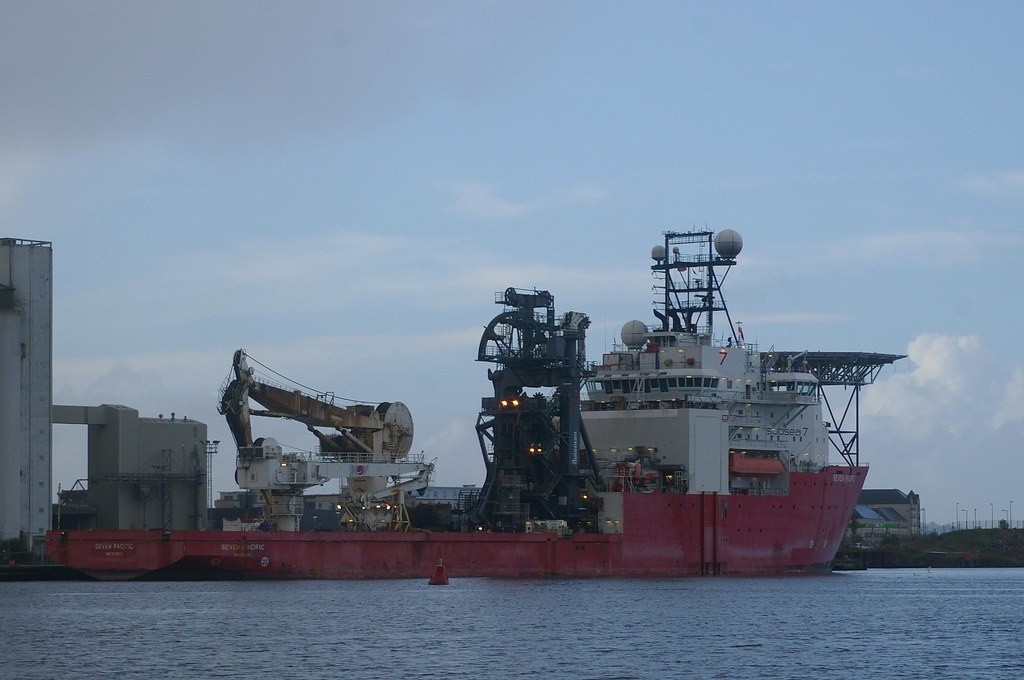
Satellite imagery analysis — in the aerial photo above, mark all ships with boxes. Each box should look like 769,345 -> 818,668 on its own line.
42,228 -> 871,580
